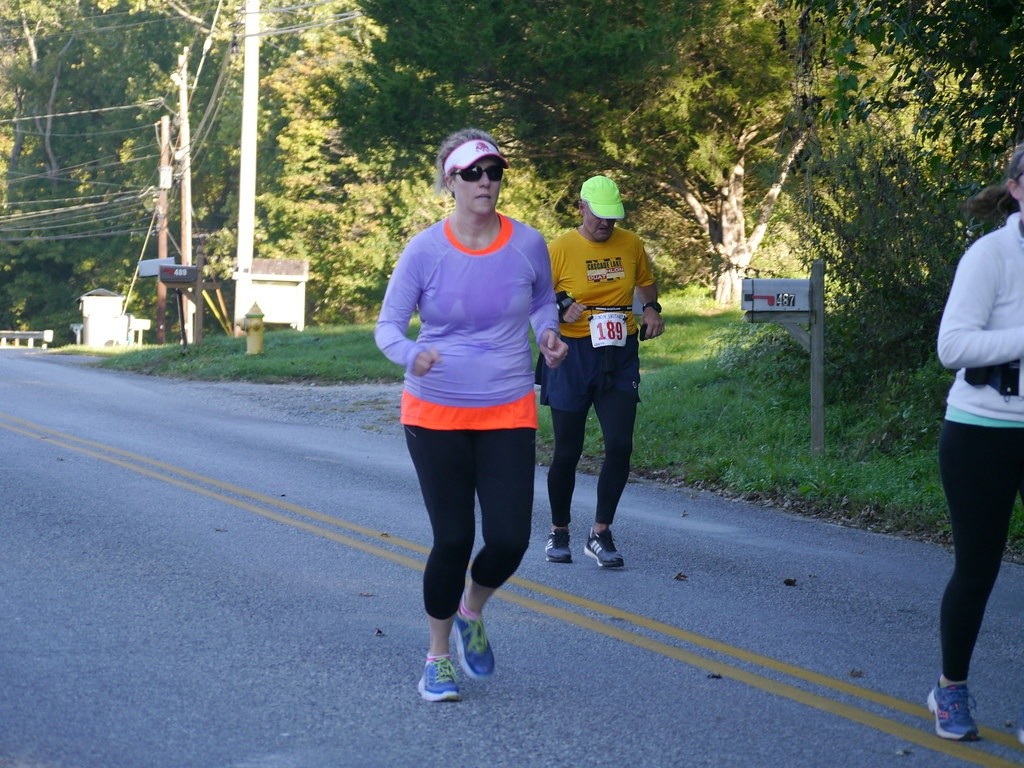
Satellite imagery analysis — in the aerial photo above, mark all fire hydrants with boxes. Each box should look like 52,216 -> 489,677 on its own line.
236,302 -> 265,354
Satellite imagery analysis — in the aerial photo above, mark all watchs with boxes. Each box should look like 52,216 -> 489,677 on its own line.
642,301 -> 662,313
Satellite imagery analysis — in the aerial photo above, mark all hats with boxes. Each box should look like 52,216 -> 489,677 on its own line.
581,175 -> 625,219
444,139 -> 509,180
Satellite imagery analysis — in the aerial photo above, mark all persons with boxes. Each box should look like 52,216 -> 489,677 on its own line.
375,128 -> 567,700
535,174 -> 666,568
925,145 -> 1024,738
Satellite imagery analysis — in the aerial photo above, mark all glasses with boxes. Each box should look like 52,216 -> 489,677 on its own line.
450,165 -> 503,182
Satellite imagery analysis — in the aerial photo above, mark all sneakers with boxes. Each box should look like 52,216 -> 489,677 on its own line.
927,679 -> 978,739
583,526 -> 625,569
416,660 -> 461,702
454,609 -> 495,680
545,527 -> 572,563
